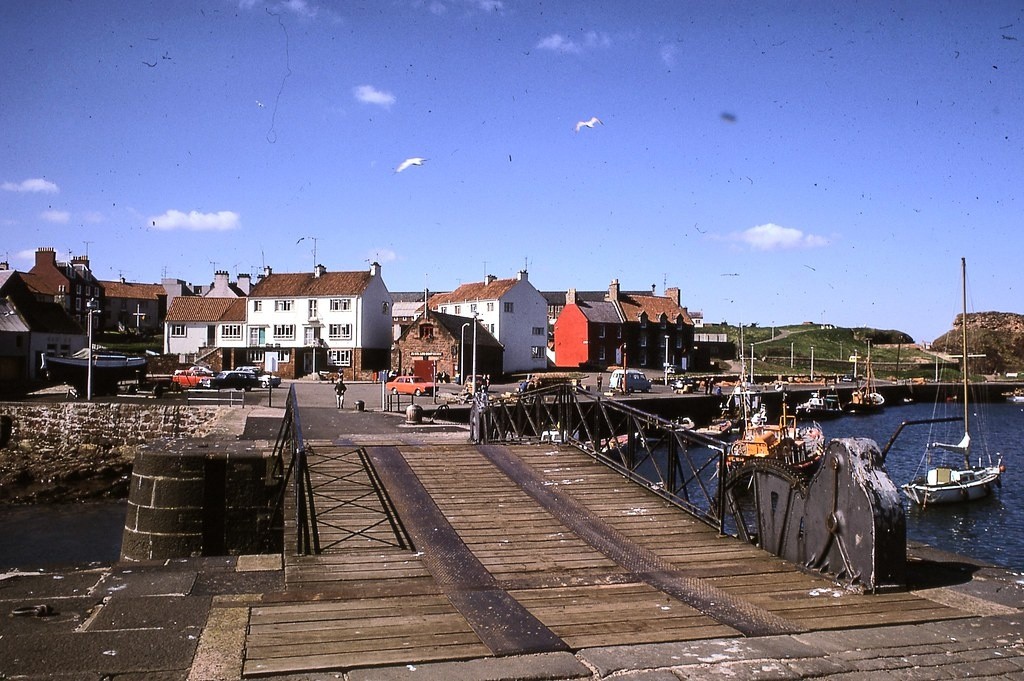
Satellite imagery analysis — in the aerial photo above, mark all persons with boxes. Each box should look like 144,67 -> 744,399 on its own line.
704,376 -> 725,396
337,367 -> 345,382
436,370 -> 446,383
595,372 -> 603,393
333,379 -> 347,410
401,365 -> 414,376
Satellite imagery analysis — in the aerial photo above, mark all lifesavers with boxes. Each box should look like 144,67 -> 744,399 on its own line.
983,484 -> 992,493
994,478 -> 1002,488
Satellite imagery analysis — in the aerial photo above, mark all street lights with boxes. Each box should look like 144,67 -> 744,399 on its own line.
86,308 -> 101,401
460,322 -> 471,385
664,335 -> 670,385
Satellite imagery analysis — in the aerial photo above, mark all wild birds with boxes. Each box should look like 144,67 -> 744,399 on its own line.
576,116 -> 604,133
396,157 -> 427,174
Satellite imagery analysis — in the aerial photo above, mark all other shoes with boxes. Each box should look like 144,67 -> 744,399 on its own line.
337,406 -> 340,409
341,405 -> 343,409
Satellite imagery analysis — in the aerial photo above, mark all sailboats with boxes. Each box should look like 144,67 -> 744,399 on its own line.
900,256 -> 1007,509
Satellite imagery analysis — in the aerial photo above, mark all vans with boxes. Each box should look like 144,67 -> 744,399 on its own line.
608,368 -> 652,394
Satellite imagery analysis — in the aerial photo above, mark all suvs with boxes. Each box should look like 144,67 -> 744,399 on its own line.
143,364 -> 282,392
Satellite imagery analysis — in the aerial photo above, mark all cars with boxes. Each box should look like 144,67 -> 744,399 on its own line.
464,374 -> 488,392
385,375 -> 439,397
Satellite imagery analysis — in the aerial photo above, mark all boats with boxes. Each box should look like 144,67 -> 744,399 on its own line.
795,383 -> 844,421
715,320 -> 767,436
583,425 -> 646,460
715,391 -> 826,496
663,416 -> 695,438
1000,387 -> 1024,406
847,338 -> 886,414
689,420 -> 732,444
